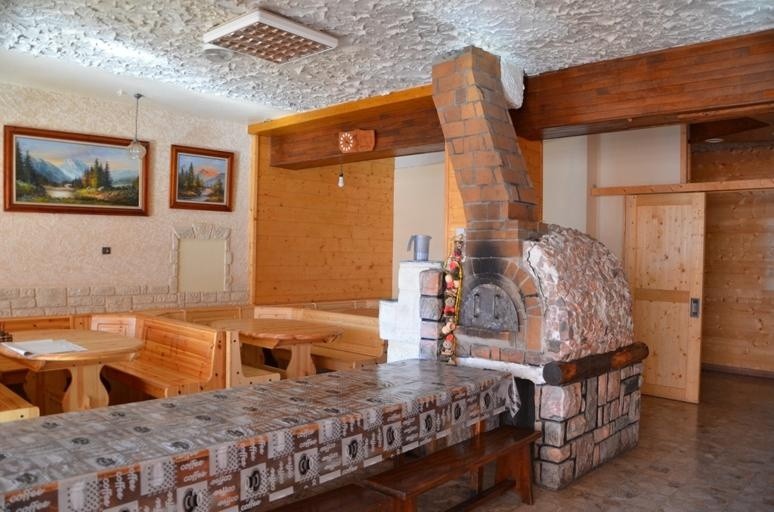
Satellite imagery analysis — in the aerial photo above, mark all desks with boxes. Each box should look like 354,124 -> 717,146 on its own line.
0,359 -> 522,511
1,329 -> 144,412
215,319 -> 345,379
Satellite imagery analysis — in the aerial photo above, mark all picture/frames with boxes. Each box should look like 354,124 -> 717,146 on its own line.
4,125 -> 149,216
170,144 -> 234,212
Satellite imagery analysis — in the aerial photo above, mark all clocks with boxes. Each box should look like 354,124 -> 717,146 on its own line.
338,129 -> 375,153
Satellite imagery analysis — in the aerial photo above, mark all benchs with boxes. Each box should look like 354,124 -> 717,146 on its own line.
269,484 -> 395,512
0,384 -> 39,422
226,330 -> 280,388
363,426 -> 542,512
90,318 -> 226,399
269,327 -> 387,371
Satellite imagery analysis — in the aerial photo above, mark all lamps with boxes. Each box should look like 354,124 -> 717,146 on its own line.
127,94 -> 146,160
202,8 -> 338,67
338,156 -> 344,188
